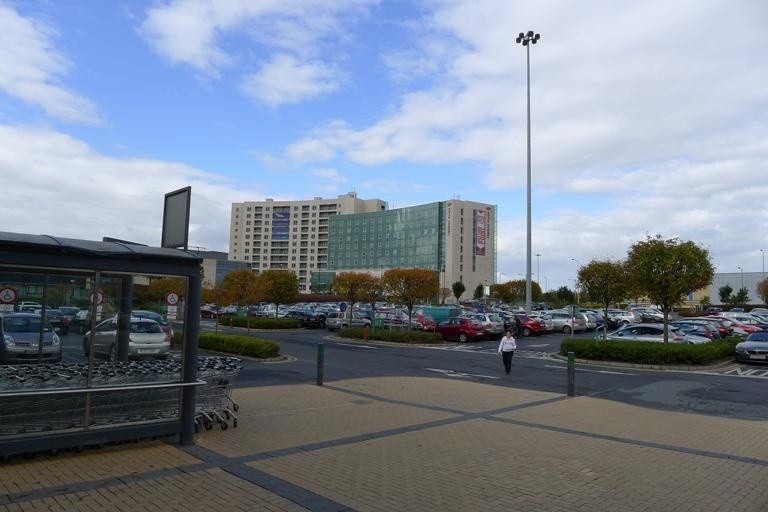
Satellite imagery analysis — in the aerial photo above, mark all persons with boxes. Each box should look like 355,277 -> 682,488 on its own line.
497,329 -> 517,375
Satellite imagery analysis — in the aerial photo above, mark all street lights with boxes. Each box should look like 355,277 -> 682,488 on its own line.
738,266 -> 743,289
760,248 -> 765,273
516,30 -> 540,315
535,253 -> 541,285
568,258 -> 580,304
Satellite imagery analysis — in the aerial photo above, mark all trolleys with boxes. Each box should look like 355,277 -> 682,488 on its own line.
0,355 -> 244,455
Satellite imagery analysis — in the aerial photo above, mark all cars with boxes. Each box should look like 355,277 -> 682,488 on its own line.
198,302 -> 673,342
17,301 -> 108,333
594,322 -> 712,342
0,310 -> 62,364
83,316 -> 171,359
113,310 -> 174,348
669,305 -> 768,340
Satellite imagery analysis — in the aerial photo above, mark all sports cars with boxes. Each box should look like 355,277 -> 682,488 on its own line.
735,331 -> 768,363
